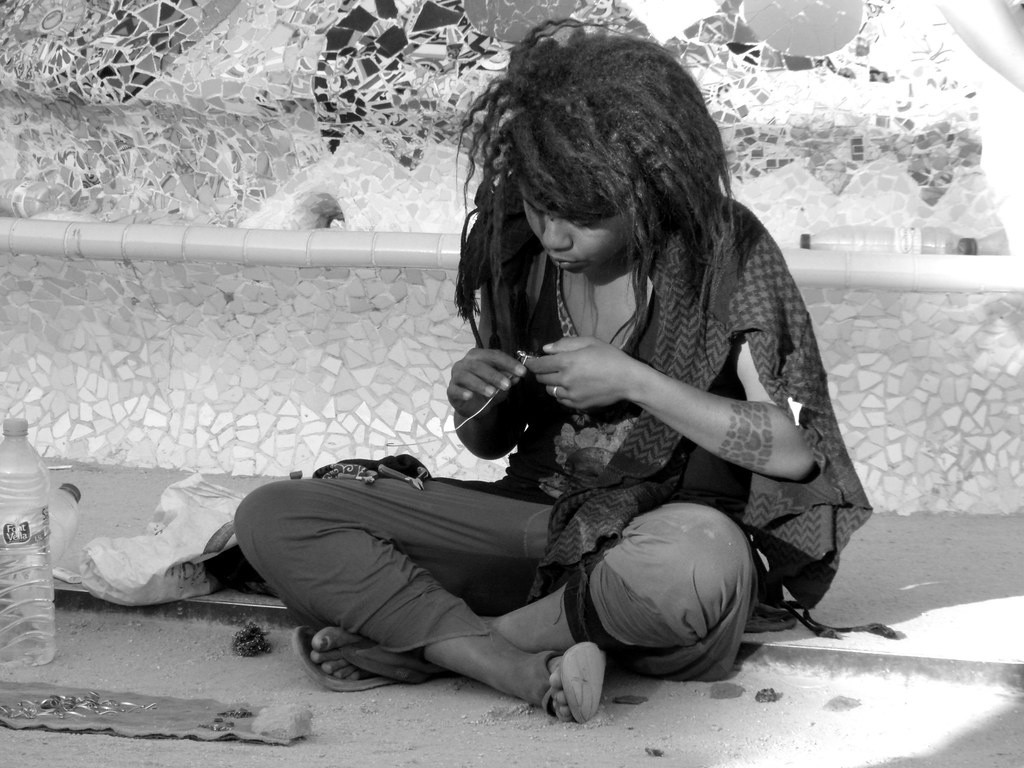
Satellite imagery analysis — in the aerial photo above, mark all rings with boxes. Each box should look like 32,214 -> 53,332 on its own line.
554,387 -> 558,398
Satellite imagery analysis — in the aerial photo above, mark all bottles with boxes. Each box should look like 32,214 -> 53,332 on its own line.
799,225 -> 914,254
50,482 -> 81,565
914,225 -> 979,256
0,417 -> 56,667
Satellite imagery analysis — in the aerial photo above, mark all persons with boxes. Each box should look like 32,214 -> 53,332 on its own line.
234,18 -> 871,722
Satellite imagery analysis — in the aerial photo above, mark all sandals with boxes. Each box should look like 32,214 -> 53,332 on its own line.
541,641 -> 606,724
293,625 -> 401,692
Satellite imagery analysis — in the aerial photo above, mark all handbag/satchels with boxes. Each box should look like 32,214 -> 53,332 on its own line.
80,471 -> 247,607
